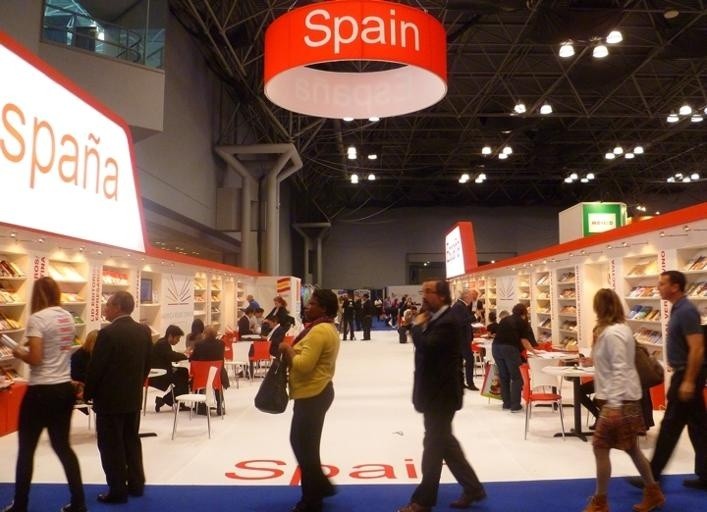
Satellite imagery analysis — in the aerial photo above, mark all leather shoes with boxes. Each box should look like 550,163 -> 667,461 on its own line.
320,479 -> 336,498
287,496 -> 323,512
463,384 -> 468,388
126,485 -> 144,498
447,485 -> 486,509
396,500 -> 433,512
96,492 -> 128,504
624,477 -> 661,489
178,402 -> 190,411
468,383 -> 479,391
683,477 -> 707,490
217,406 -> 225,415
155,396 -> 165,413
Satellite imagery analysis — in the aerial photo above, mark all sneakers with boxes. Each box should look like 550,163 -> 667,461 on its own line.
60,504 -> 87,512
0,500 -> 27,512
511,405 -> 523,413
589,419 -> 598,429
503,403 -> 510,410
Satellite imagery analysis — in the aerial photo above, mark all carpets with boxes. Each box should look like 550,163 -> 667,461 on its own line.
1,474 -> 707,512
337,314 -> 398,330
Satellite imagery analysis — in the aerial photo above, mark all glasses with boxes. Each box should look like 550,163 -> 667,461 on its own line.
417,288 -> 438,297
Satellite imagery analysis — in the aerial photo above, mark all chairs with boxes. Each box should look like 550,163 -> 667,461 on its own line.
470,325 -> 665,449
70,330 -> 295,441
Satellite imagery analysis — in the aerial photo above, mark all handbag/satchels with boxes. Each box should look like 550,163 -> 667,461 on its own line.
254,349 -> 289,414
633,334 -> 664,389
220,368 -> 230,390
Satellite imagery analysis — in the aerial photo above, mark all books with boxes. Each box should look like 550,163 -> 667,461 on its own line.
0,256 -> 129,388
522,252 -> 707,374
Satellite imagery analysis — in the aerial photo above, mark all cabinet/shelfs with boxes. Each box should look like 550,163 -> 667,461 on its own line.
354,289 -> 371,308
445,201 -> 707,409
0,220 -> 265,438
331,289 -> 348,325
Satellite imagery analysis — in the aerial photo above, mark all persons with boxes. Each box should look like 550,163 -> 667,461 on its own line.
399,277 -> 486,509
624,271 -> 707,491
574,382 -> 601,431
82,291 -> 154,505
278,290 -> 340,510
583,288 -> 665,512
148,291 -> 539,418
5,277 -> 86,510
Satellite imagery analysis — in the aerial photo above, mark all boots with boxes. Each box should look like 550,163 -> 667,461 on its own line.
582,492 -> 610,512
632,481 -> 666,511
